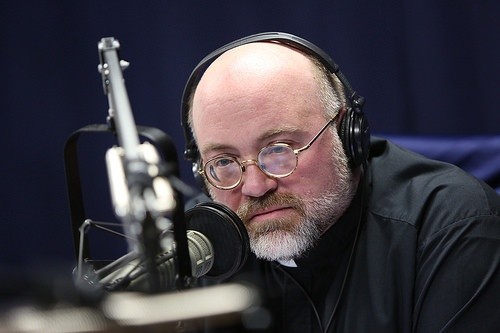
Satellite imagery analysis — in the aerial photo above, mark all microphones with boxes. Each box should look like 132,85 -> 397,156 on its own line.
99,230 -> 215,292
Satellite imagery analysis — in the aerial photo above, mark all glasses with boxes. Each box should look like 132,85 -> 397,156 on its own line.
198,109 -> 340,191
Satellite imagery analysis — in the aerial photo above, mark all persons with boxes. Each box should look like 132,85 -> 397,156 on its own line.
179,31 -> 500,333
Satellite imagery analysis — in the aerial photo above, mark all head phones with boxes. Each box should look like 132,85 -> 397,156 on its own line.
180,33 -> 373,170
